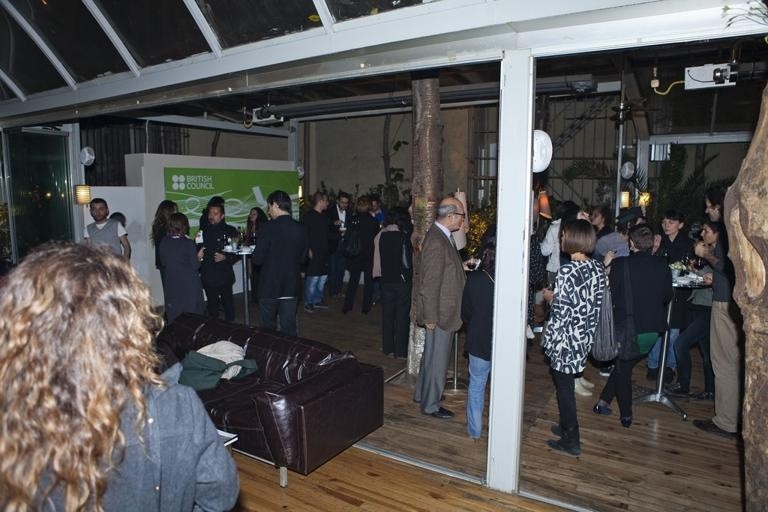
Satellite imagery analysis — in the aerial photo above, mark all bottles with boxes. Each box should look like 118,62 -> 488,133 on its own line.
220,225 -> 257,254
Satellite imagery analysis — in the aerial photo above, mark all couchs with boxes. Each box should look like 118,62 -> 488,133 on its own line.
161,308 -> 386,479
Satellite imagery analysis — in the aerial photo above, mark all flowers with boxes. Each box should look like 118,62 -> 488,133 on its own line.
667,260 -> 687,269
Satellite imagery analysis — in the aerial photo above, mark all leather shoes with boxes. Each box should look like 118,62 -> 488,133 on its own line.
593,404 -> 632,427
413,394 -> 454,418
547,425 -> 581,457
693,419 -> 740,438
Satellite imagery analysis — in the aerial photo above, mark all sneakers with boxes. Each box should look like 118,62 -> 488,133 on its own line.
599,366 -> 614,376
664,382 -> 715,400
304,301 -> 328,312
574,376 -> 594,396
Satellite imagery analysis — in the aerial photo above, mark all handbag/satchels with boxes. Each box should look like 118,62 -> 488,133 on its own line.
591,283 -> 639,362
178,349 -> 226,390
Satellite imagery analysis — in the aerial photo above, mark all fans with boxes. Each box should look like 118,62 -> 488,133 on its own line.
584,87 -> 661,123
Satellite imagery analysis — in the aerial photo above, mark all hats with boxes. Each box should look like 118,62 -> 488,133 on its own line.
615,206 -> 648,224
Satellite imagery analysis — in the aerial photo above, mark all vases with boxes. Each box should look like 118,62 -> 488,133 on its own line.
672,268 -> 681,278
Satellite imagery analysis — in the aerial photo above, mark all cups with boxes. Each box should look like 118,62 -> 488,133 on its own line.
466,249 -> 478,271
671,253 -> 710,286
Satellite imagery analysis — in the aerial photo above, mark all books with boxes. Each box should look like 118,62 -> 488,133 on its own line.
216,427 -> 240,448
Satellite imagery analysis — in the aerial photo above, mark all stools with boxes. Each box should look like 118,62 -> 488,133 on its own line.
443,326 -> 470,395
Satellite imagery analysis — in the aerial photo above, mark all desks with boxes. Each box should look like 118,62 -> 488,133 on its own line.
631,273 -> 714,422
194,234 -> 282,327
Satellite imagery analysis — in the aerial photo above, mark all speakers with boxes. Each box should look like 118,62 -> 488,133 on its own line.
296,167 -> 304,180
531,129 -> 553,173
621,162 -> 635,179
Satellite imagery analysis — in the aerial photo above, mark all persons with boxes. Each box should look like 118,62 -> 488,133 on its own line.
0,241 -> 240,510
153,187 -> 498,438
533,189 -> 743,455
84,198 -> 132,261
109,212 -> 127,230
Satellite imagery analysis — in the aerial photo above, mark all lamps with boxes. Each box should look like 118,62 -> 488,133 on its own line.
531,127 -> 553,178
616,153 -> 638,181
533,187 -> 553,225
74,182 -> 91,206
